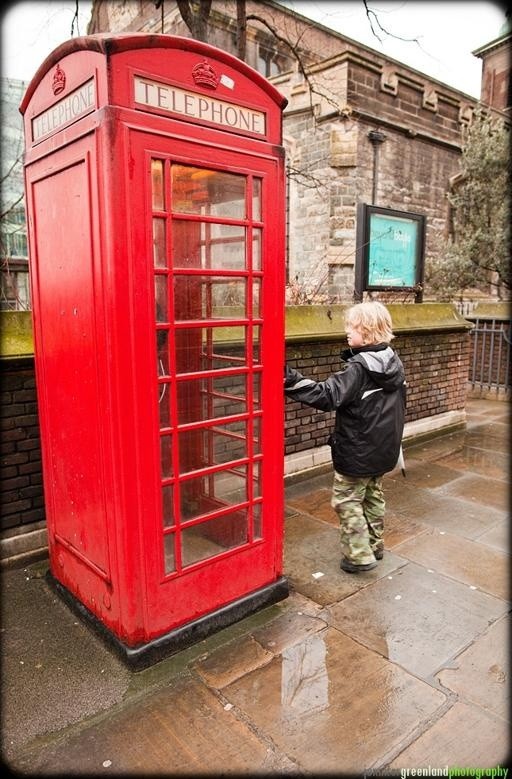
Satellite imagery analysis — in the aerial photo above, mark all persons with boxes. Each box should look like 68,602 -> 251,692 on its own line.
285,299 -> 408,576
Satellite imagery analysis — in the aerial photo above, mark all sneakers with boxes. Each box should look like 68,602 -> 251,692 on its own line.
340,552 -> 383,572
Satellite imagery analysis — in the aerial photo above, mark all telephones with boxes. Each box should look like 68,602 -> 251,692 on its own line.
157,304 -> 168,354
